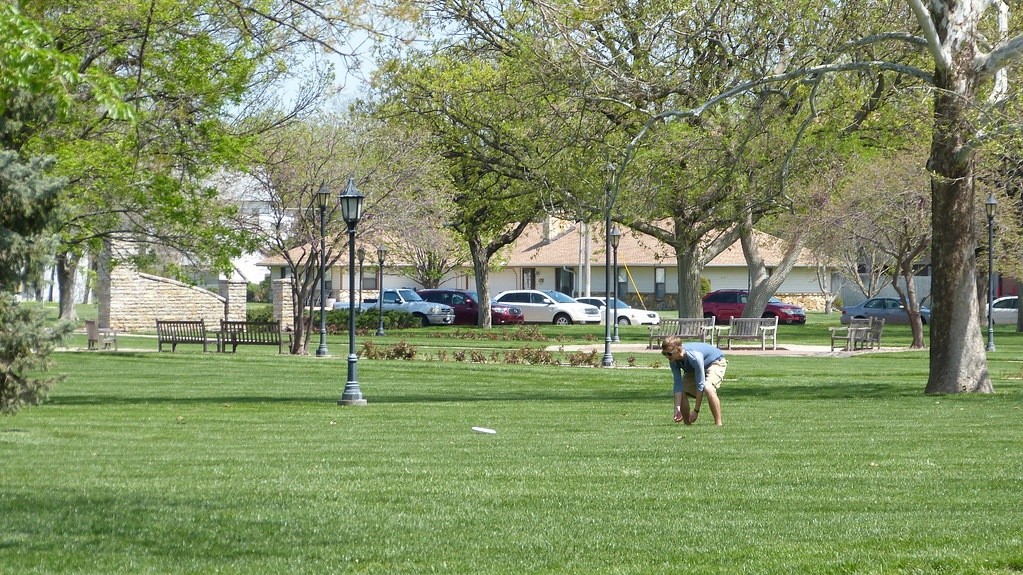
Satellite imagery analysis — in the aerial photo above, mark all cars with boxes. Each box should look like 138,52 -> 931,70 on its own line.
840,297 -> 931,326
985,296 -> 1019,325
574,297 -> 660,326
494,290 -> 601,325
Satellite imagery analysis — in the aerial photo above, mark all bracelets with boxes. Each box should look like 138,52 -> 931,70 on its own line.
694,408 -> 700,413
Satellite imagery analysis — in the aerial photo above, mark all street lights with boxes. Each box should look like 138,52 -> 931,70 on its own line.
316,180 -> 330,357
600,164 -> 615,368
357,241 -> 367,314
611,224 -> 621,343
336,178 -> 367,407
375,242 -> 387,336
984,191 -> 997,352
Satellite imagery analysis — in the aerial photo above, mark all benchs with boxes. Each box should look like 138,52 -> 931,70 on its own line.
648,316 -> 716,351
155,318 -> 222,352
220,319 -> 293,355
715,315 -> 778,350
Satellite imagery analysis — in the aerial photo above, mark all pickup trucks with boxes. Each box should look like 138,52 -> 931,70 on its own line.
333,287 -> 464,327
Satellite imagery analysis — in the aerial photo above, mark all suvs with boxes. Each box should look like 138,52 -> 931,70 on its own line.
417,289 -> 524,325
702,289 -> 807,326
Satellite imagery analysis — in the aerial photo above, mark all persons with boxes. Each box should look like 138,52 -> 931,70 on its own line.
661,335 -> 728,426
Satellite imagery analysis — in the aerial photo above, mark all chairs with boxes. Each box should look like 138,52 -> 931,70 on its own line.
85,319 -> 119,351
892,303 -> 898,307
828,316 -> 885,352
453,297 -> 465,304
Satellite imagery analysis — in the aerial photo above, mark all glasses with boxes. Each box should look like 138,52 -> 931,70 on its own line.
661,347 -> 678,356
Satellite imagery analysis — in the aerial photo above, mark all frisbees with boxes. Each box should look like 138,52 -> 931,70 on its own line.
471,426 -> 496,435
